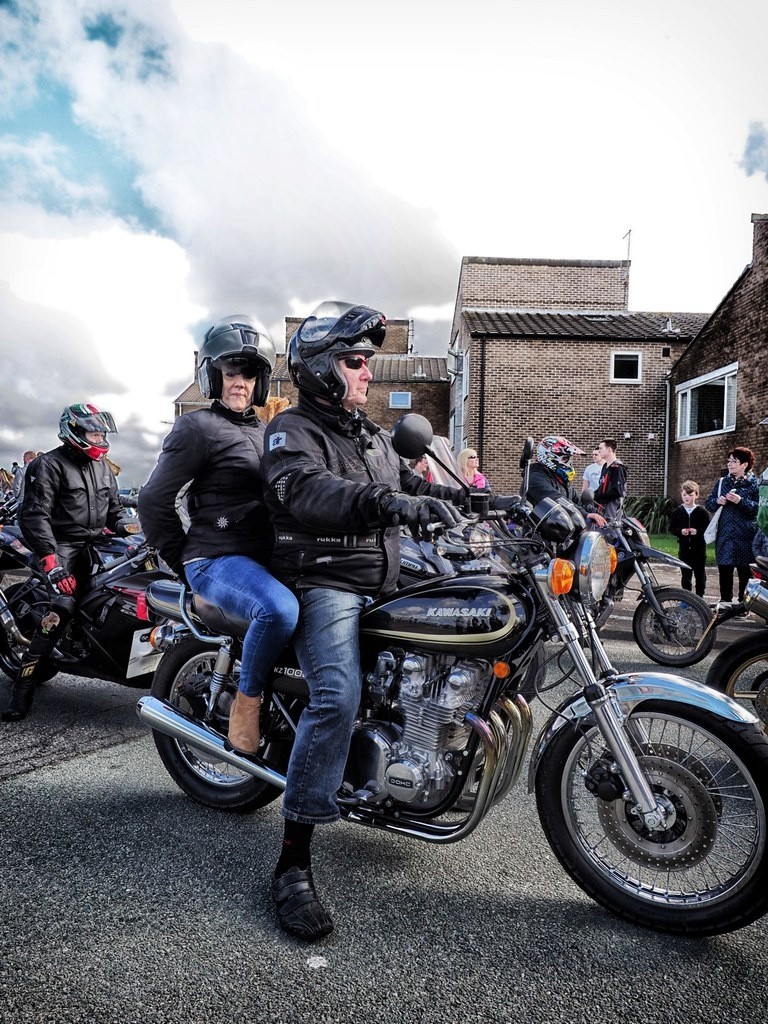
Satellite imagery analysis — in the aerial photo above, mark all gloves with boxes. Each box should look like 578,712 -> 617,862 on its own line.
39,554 -> 76,594
492,494 -> 533,510
380,489 -> 462,536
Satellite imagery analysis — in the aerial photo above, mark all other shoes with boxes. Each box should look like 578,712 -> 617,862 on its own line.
735,611 -> 750,619
679,602 -> 692,609
228,689 -> 261,755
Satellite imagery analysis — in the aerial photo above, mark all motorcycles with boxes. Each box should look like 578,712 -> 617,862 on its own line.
450,487 -> 716,668
133,414 -> 768,938
706,554 -> 768,736
0,496 -> 189,688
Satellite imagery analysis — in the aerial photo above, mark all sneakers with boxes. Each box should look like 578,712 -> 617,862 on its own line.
272,871 -> 334,940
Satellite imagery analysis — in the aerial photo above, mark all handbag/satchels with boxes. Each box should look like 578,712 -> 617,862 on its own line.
704,506 -> 722,544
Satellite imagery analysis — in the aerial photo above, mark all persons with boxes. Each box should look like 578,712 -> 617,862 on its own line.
139,315 -> 299,757
261,302 -> 534,938
0,403 -> 133,722
0,451 -> 45,526
669,447 -> 768,610
519,436 -> 629,529
406,435 -> 493,495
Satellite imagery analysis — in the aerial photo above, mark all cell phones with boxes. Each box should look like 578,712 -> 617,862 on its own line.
729,488 -> 737,493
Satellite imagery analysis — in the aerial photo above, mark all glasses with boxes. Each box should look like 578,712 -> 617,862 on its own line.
338,356 -> 369,369
468,456 -> 475,459
592,454 -> 599,456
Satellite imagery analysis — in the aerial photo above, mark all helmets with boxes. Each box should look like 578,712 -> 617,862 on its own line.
286,301 -> 386,404
197,315 -> 276,406
58,403 -> 111,462
537,436 -> 587,482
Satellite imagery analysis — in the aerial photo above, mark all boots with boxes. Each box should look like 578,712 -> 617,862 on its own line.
2,651 -> 48,721
